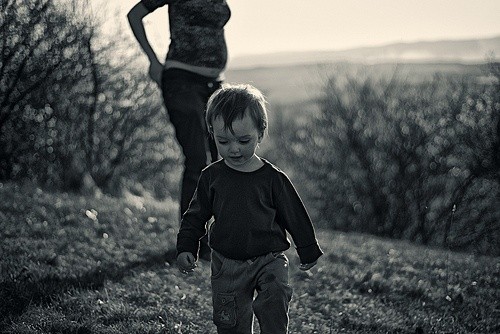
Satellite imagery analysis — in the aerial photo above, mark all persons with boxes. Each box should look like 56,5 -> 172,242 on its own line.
125,0 -> 232,264
175,85 -> 325,334
